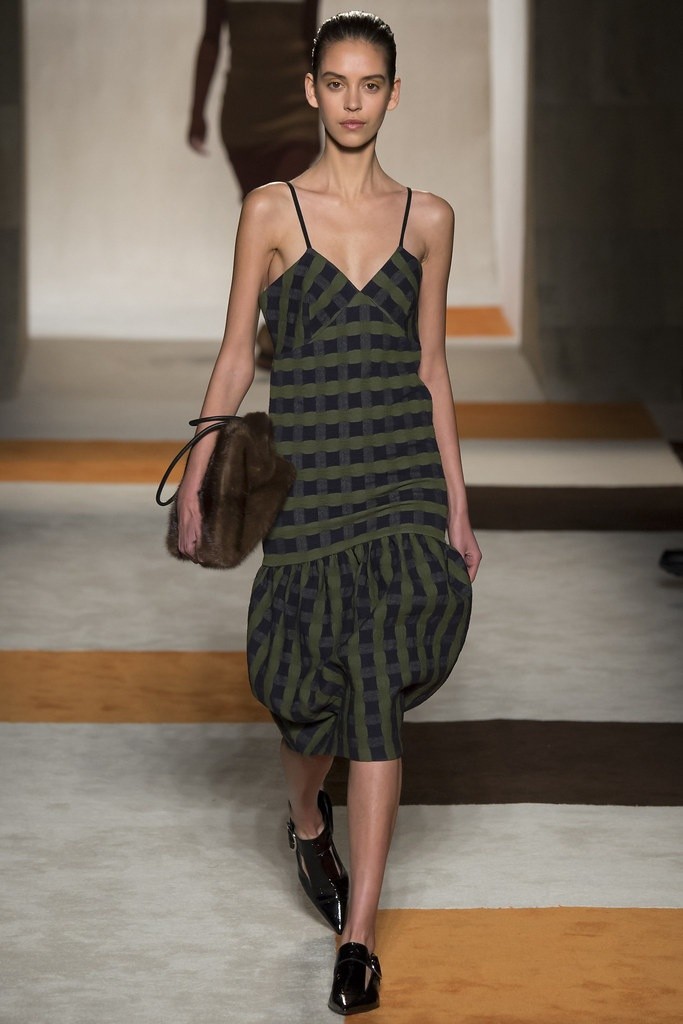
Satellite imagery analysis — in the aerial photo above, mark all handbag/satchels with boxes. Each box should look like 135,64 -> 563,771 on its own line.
153,413 -> 293,572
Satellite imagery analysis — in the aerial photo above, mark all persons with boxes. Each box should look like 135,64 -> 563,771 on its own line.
177,12 -> 482,1016
188,0 -> 321,199
660,549 -> 683,575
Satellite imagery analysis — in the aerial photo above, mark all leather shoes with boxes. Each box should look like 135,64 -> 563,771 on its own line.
328,942 -> 381,1016
287,790 -> 347,934
